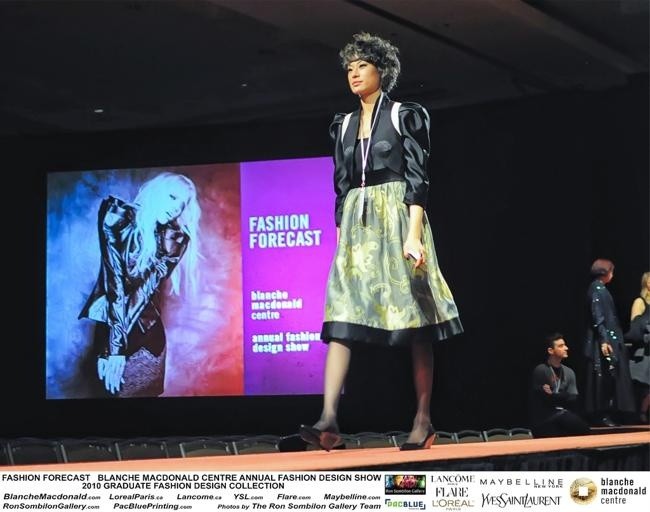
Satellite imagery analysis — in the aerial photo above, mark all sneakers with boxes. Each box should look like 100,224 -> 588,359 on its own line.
594,416 -> 621,428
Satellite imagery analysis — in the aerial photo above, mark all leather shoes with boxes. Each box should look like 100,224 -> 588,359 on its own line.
299,423 -> 339,452
399,423 -> 436,451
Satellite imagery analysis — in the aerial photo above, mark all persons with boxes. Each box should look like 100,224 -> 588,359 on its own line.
623,269 -> 650,421
529,332 -> 592,438
297,32 -> 468,450
72,172 -> 200,396
582,259 -> 636,426
